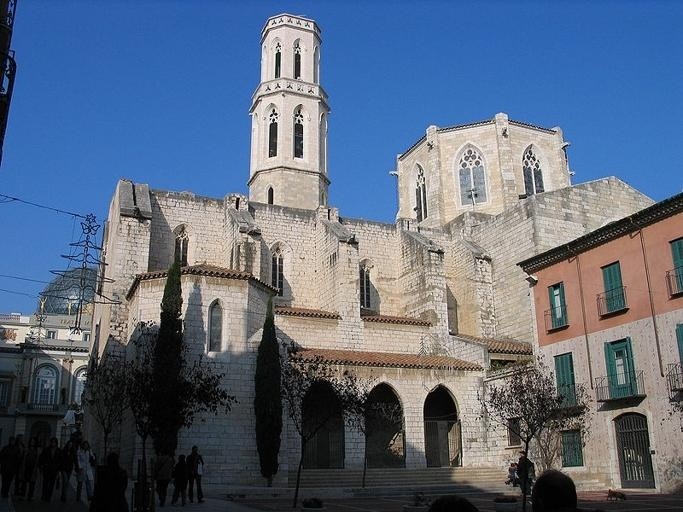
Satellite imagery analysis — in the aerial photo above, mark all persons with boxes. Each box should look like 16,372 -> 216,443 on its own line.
531,468 -> 577,511
504,450 -> 535,496
0,430 -> 205,511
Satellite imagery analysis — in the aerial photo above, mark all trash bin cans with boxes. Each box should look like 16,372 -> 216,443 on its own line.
90,467 -> 130,512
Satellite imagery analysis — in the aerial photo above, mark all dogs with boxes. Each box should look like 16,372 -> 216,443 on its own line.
607,489 -> 626,501
